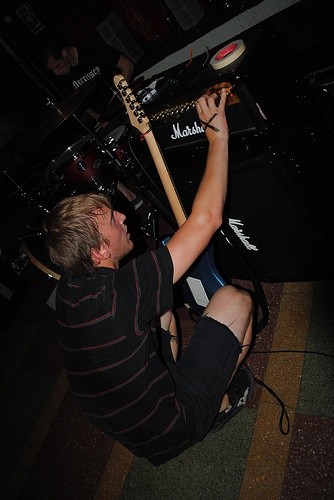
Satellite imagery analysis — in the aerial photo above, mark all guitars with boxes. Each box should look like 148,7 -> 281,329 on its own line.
112,72 -> 269,333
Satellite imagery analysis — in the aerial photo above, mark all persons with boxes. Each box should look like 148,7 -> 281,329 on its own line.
42,31 -> 134,128
43,89 -> 257,467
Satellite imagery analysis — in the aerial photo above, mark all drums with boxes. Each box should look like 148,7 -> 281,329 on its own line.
48,135 -> 117,196
2,223 -> 62,303
100,126 -> 146,172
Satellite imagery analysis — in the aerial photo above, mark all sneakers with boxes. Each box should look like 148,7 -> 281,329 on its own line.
211,368 -> 254,433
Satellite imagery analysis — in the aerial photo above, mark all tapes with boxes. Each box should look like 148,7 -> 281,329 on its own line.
210,40 -> 245,69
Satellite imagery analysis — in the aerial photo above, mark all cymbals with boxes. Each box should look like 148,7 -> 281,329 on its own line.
0,160 -> 42,204
0,160 -> 13,183
28,78 -> 97,146
